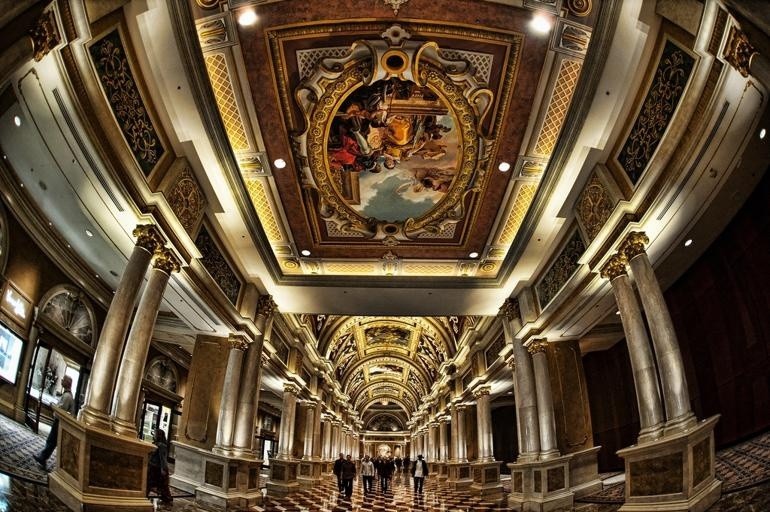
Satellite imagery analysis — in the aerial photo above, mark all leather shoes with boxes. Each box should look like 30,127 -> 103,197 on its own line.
33,454 -> 46,468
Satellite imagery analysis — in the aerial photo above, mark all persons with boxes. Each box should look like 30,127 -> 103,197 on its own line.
145,427 -> 174,506
358,452 -> 411,493
331,79 -> 453,192
411,454 -> 428,494
340,454 -> 356,498
333,453 -> 347,492
34,376 -> 77,466
